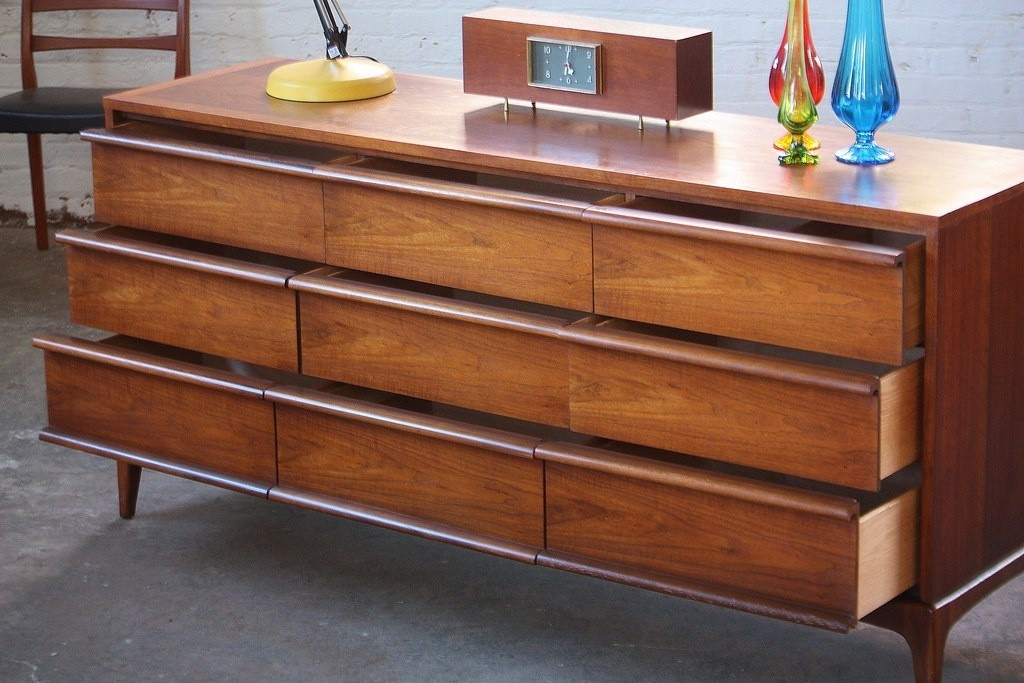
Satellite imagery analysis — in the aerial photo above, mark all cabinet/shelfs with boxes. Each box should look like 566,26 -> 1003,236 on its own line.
32,55 -> 1023,683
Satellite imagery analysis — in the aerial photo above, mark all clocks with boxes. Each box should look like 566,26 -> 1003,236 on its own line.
462,5 -> 713,130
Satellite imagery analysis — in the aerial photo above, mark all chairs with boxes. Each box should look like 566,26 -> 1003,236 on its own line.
0,0 -> 191,249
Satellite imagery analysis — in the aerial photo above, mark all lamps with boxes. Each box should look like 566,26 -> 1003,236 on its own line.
266,0 -> 398,100
